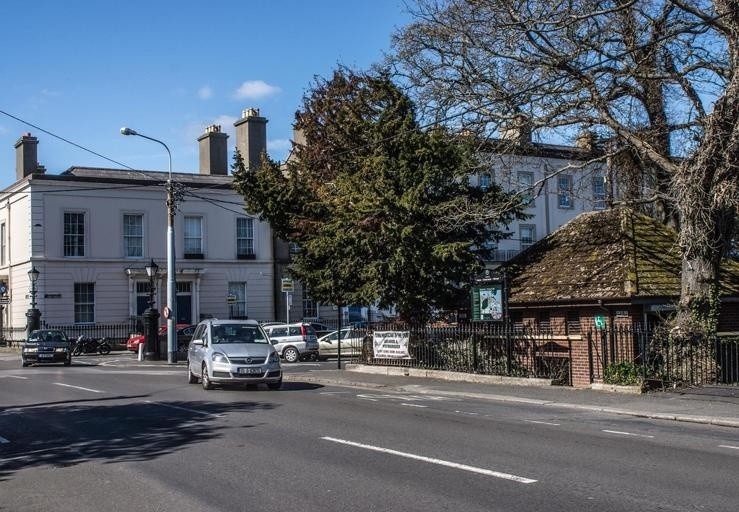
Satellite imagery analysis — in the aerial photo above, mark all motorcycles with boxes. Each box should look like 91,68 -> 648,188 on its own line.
73,334 -> 111,357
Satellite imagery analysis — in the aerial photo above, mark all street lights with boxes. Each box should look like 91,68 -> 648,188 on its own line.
120,126 -> 177,364
26,266 -> 43,338
143,257 -> 160,360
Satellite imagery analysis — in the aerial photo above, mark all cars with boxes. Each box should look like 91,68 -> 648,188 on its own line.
127,324 -> 195,353
317,328 -> 367,359
22,329 -> 71,369
309,321 -> 335,330
261,321 -> 287,327
159,326 -> 197,353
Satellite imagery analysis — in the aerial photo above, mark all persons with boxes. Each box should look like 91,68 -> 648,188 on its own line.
213,328 -> 227,342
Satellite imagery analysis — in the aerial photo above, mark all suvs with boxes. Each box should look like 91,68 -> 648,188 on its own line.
261,323 -> 319,362
187,315 -> 282,389
